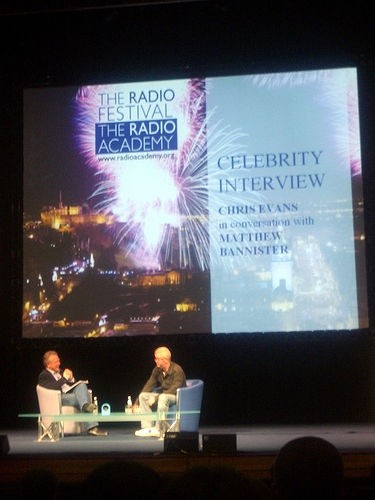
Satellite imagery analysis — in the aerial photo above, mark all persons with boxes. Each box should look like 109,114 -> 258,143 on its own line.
131,347 -> 188,437
38,350 -> 109,437
8,437 -> 375,500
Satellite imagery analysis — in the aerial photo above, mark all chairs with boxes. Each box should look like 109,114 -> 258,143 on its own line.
151,379 -> 204,433
36,383 -> 94,434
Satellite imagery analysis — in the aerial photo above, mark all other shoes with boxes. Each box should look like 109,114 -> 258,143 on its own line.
135,428 -> 152,436
83,403 -> 96,413
148,430 -> 165,437
87,429 -> 108,436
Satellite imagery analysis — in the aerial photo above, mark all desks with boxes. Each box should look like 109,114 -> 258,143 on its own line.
17,411 -> 174,442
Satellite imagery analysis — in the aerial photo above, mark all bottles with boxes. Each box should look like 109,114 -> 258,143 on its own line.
127,396 -> 132,414
93,397 -> 98,414
